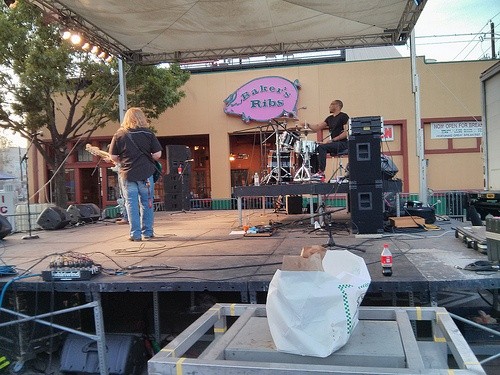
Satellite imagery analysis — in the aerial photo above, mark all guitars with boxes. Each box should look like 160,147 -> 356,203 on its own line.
85,143 -> 162,183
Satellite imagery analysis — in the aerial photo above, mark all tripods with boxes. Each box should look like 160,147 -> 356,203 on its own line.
269,208 -> 365,252
259,119 -> 313,186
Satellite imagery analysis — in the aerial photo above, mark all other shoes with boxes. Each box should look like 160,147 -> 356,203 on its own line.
312,170 -> 325,178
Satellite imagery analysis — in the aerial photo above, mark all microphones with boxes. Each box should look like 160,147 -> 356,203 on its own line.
302,106 -> 307,109
31,131 -> 44,136
107,144 -> 110,148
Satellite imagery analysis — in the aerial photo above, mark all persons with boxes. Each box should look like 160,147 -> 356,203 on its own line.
109,107 -> 162,242
301,100 -> 349,182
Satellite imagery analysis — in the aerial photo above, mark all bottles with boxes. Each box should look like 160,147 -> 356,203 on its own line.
253,172 -> 259,186
380,243 -> 393,278
189,190 -> 199,199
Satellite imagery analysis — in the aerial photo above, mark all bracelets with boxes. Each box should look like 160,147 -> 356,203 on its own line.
332,139 -> 335,142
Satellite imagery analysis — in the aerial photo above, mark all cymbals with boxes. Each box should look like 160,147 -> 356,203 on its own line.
271,117 -> 300,122
288,125 -> 303,130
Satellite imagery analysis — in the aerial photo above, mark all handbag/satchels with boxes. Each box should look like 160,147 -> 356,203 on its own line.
266,249 -> 372,358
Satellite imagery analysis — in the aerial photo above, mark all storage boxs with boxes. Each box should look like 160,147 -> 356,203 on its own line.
389,215 -> 426,229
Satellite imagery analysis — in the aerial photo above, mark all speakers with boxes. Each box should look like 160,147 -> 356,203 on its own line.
164,174 -> 190,211
37,206 -> 71,230
348,184 -> 384,234
166,145 -> 191,176
60,334 -> 146,375
381,156 -> 399,180
348,134 -> 382,185
67,203 -> 101,225
0,214 -> 12,240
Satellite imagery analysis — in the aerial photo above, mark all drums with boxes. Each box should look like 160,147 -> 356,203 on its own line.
280,130 -> 298,149
293,140 -> 316,155
